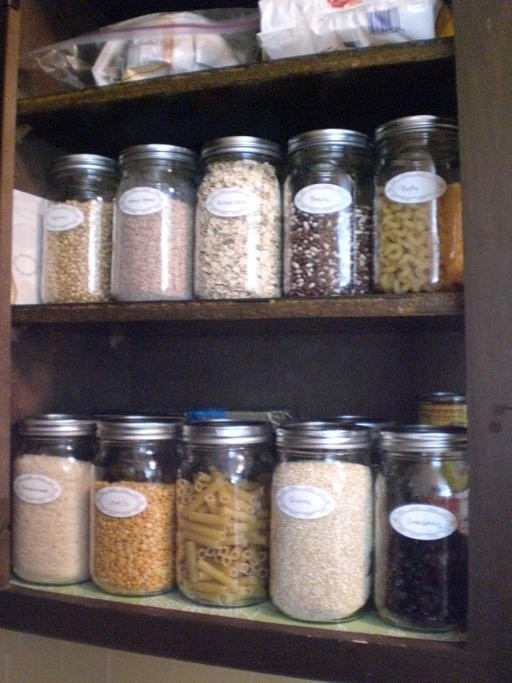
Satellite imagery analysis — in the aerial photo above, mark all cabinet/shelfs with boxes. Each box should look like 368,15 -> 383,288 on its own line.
1,0 -> 509,680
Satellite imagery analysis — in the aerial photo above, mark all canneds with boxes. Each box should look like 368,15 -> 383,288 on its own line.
285,127 -> 371,296
39,150 -> 110,305
270,388 -> 467,631
94,414 -> 175,597
194,135 -> 284,301
373,117 -> 465,293
175,403 -> 291,608
109,143 -> 194,301
12,409 -> 94,588
